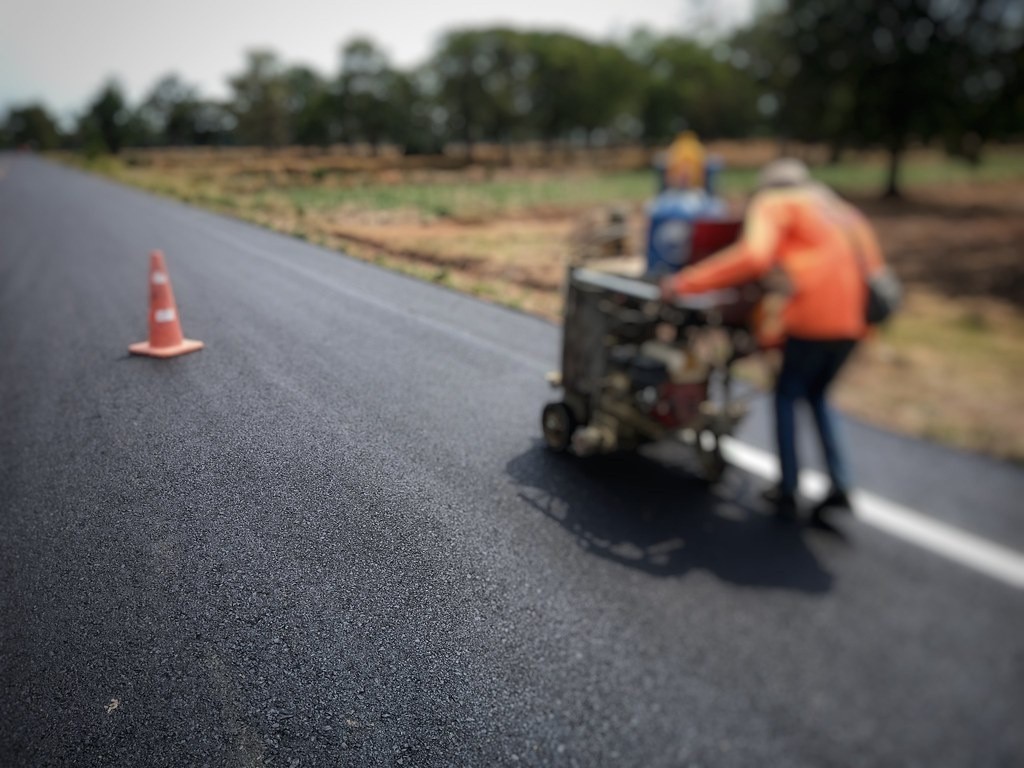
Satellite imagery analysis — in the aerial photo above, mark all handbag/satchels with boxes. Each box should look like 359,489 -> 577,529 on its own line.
865,281 -> 890,326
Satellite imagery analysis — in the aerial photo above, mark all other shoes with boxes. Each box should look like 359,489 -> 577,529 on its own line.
810,489 -> 848,512
760,485 -> 796,510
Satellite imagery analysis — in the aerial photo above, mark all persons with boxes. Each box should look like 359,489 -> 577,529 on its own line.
662,160 -> 884,517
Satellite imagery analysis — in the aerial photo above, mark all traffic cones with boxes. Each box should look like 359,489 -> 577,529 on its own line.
127,249 -> 206,360
